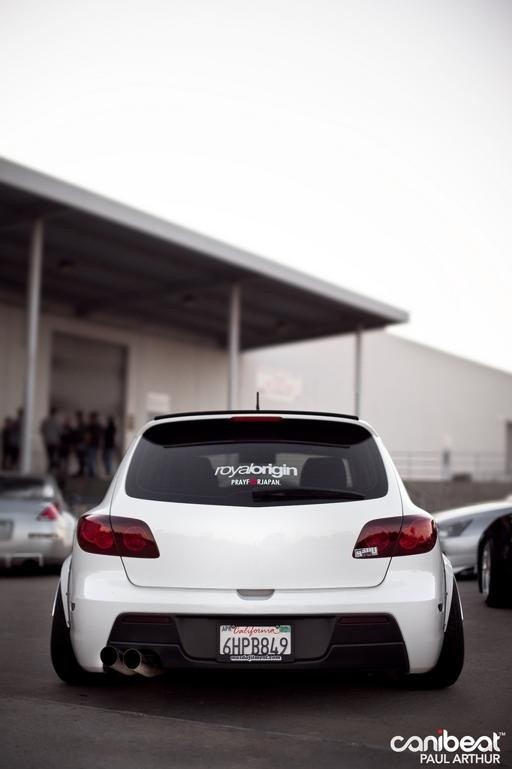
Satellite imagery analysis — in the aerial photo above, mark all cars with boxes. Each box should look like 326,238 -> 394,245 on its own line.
48,388 -> 468,700
0,471 -> 84,570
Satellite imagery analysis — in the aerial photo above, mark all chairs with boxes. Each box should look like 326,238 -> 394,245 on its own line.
173,456 -> 346,500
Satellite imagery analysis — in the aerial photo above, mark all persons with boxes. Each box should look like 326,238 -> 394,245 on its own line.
1,407 -> 22,468
43,406 -> 116,477
